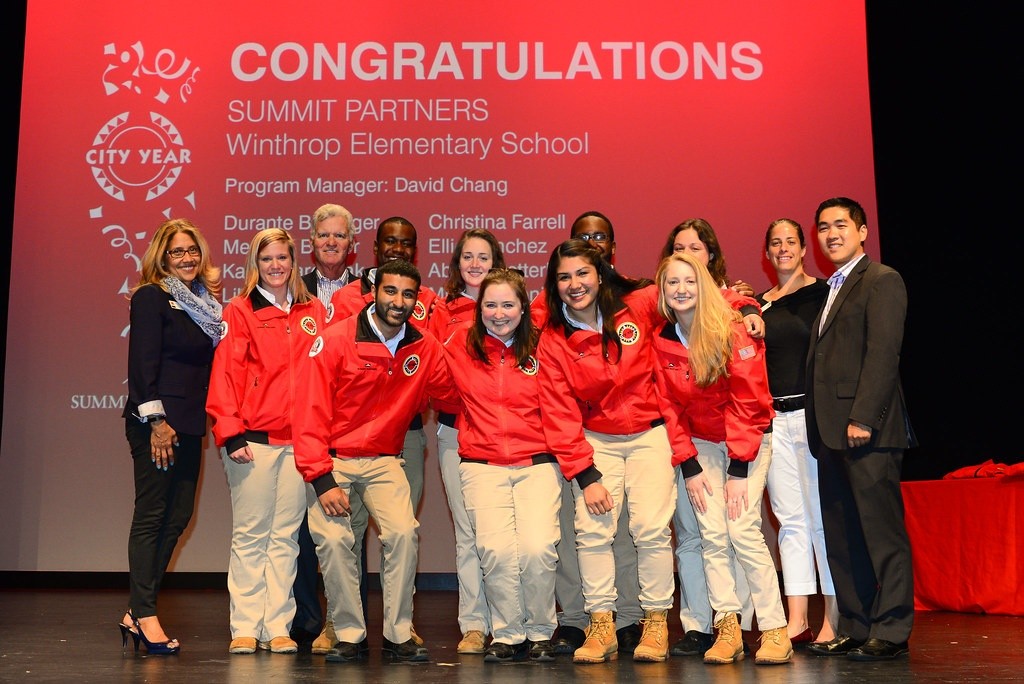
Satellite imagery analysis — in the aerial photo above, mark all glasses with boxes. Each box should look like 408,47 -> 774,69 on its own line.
163,247 -> 201,258
575,233 -> 611,243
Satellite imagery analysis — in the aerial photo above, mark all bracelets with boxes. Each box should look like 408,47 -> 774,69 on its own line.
148,415 -> 165,422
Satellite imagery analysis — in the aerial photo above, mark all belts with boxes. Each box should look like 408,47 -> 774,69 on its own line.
773,395 -> 805,413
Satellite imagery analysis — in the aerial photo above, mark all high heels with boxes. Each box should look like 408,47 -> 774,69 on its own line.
119,612 -> 136,648
130,618 -> 180,656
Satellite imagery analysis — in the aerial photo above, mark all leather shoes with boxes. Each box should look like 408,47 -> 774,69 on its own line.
756,628 -> 812,645
846,638 -> 910,660
809,636 -> 862,655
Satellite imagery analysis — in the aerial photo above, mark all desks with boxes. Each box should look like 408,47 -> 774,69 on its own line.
901,474 -> 1024,613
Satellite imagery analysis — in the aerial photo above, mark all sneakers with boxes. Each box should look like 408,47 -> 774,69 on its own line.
312,625 -> 339,654
702,612 -> 744,664
673,629 -> 714,655
615,623 -> 642,654
381,636 -> 429,661
326,635 -> 368,662
482,642 -> 524,662
229,637 -> 256,653
409,623 -> 423,647
553,626 -> 588,654
742,639 -> 750,655
457,629 -> 486,653
632,608 -> 670,662
754,625 -> 794,663
525,638 -> 556,661
572,612 -> 618,663
259,635 -> 298,653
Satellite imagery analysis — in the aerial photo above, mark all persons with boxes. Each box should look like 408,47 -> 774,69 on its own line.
290,262 -> 460,666
803,195 -> 920,661
651,218 -> 839,665
427,227 -> 508,658
205,227 -> 328,652
442,210 -> 756,667
289,204 -> 368,655
118,218 -> 222,656
311,216 -> 427,653
